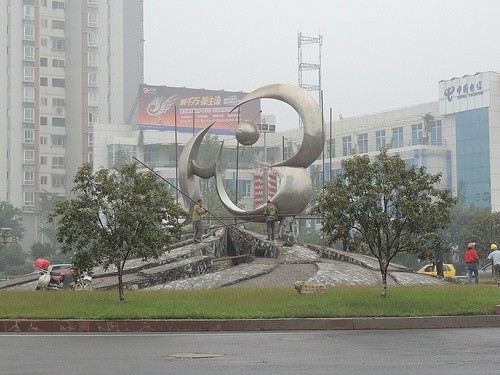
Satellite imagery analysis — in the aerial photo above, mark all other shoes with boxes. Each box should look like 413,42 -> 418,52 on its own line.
271,237 -> 274,240
266,238 -> 270,240
195,238 -> 201,243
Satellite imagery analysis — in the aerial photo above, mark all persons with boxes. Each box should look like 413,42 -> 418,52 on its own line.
465,242 -> 479,285
192,199 -> 208,243
482,244 -> 500,287
263,200 -> 278,241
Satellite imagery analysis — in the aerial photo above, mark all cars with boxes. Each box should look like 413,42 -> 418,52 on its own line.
466,262 -> 496,278
415,263 -> 456,280
45,263 -> 79,291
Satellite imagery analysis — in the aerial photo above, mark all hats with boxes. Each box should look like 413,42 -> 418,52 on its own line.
468,242 -> 475,248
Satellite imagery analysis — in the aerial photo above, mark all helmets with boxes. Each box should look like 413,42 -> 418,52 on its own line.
491,244 -> 496,249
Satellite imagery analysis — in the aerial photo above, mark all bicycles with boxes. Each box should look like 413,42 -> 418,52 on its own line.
75,275 -> 92,292
